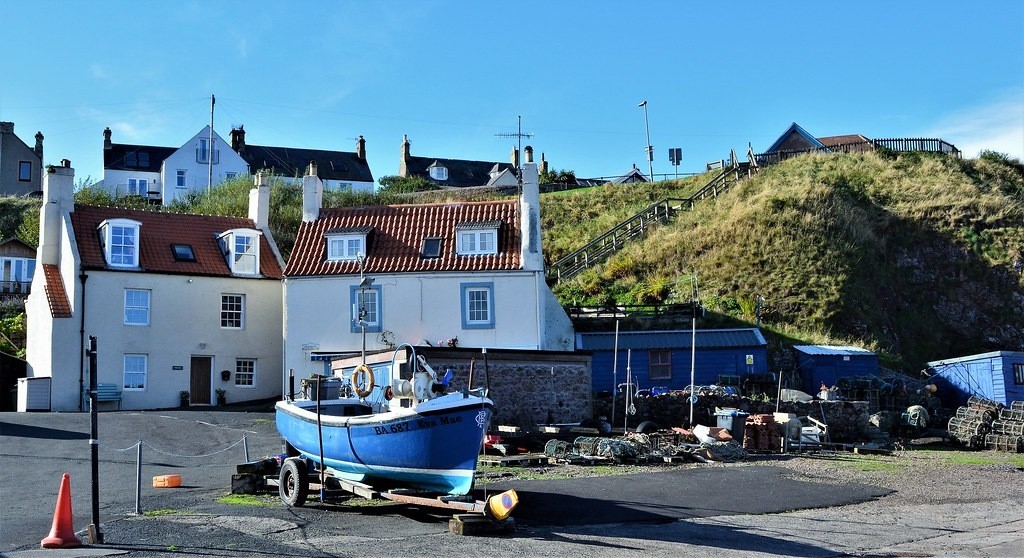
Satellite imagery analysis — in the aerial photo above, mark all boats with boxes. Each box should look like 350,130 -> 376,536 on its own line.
274,251 -> 493,499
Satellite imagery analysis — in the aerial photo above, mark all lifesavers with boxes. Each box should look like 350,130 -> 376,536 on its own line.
351,364 -> 374,397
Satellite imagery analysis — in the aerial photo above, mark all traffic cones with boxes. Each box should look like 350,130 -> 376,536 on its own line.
40,473 -> 85,548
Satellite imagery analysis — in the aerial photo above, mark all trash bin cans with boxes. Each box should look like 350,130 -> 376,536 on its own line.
713,409 -> 750,447
669,384 -> 741,395
742,371 -> 776,397
652,386 -> 668,395
635,390 -> 652,396
719,372 -> 741,384
618,382 -> 634,394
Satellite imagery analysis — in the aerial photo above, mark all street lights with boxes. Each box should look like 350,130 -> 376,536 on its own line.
639,100 -> 655,183
